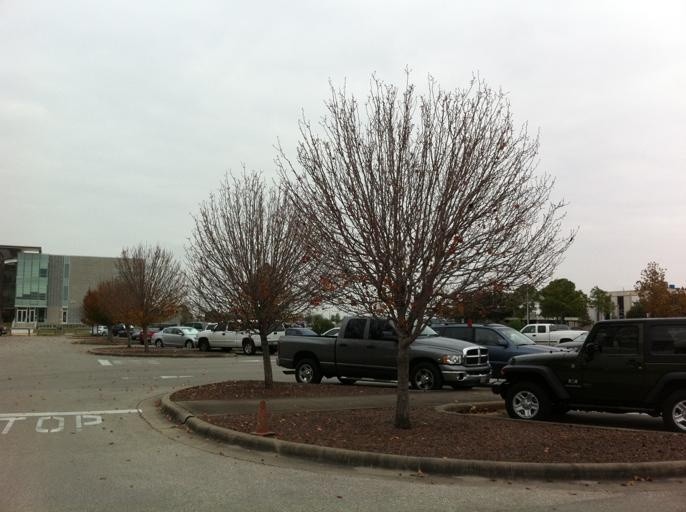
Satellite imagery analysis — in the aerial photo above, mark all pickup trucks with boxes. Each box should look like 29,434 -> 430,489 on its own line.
275,311 -> 494,390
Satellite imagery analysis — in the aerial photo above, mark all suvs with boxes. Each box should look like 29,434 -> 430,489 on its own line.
438,319 -> 575,380
489,315 -> 686,434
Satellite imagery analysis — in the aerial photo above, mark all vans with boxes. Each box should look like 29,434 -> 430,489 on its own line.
185,321 -> 205,331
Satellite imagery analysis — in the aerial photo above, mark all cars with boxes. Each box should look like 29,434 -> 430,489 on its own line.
554,331 -> 590,347
321,326 -> 341,337
150,325 -> 200,348
89,321 -> 158,345
285,326 -> 319,336
193,318 -> 287,355
519,322 -> 589,345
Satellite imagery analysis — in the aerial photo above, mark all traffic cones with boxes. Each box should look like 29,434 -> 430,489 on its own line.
248,399 -> 276,438
